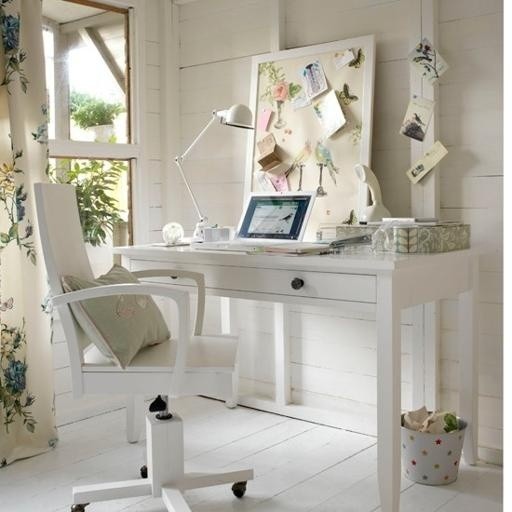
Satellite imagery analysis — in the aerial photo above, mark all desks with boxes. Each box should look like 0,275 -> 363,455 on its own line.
106,235 -> 478,512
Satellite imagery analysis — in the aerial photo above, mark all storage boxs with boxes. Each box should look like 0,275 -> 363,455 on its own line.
334,220 -> 473,256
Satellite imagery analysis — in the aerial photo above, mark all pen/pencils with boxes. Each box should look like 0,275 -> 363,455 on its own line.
314,250 -> 341,256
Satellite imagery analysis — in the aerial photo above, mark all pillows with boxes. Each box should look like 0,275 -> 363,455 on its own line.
57,259 -> 173,373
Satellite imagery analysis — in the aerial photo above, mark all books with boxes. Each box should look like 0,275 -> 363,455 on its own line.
381,216 -> 439,224
261,240 -> 332,256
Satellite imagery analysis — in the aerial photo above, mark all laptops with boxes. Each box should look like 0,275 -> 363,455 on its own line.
189,191 -> 316,251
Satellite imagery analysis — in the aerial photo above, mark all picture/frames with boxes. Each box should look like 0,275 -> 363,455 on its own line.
242,34 -> 378,245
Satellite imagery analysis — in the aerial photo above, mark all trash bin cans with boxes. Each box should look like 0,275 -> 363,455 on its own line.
401,410 -> 467,487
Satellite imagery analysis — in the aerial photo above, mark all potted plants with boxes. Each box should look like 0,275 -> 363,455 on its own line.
71,101 -> 127,142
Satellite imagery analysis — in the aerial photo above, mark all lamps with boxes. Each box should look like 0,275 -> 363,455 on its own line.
175,100 -> 254,244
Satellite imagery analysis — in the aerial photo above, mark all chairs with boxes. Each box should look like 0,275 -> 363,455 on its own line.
30,179 -> 257,512
